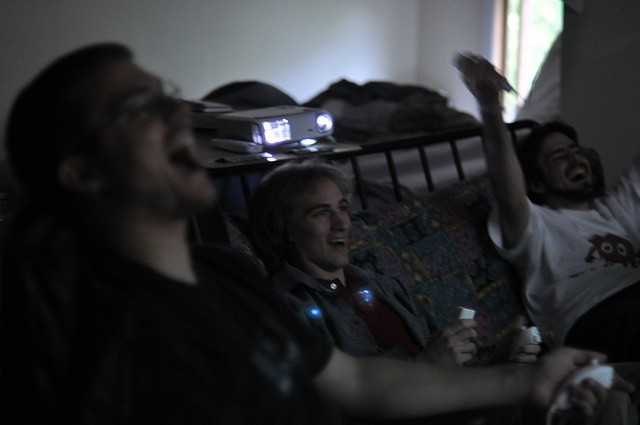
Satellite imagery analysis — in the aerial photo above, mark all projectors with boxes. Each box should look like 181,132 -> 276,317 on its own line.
213,105 -> 336,149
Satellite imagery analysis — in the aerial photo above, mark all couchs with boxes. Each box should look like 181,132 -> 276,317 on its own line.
190,118 -> 607,361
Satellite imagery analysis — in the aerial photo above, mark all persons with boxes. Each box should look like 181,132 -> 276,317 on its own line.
0,43 -> 640,425
251,159 -> 640,425
456,54 -> 640,364
